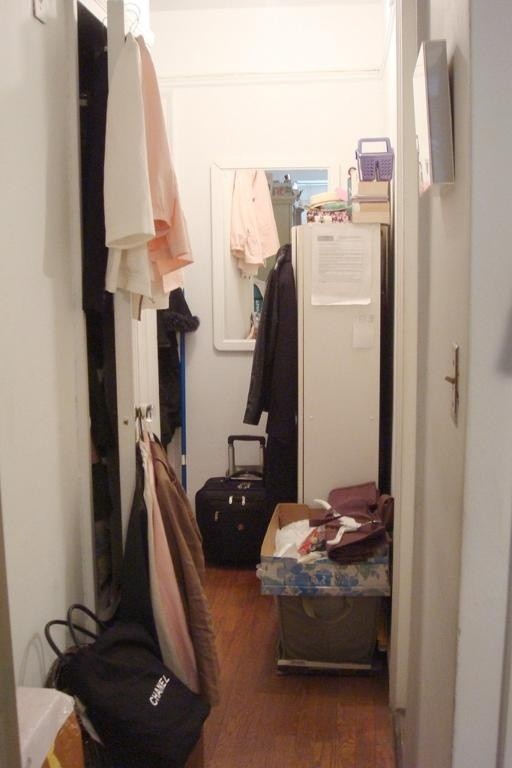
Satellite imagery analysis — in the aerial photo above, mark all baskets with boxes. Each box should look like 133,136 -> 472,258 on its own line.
355,135 -> 393,183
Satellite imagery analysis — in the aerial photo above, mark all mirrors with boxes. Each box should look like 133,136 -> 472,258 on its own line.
211,160 -> 340,352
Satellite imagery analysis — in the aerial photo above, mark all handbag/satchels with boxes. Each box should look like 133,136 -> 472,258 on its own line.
40,601 -> 212,768
324,481 -> 395,564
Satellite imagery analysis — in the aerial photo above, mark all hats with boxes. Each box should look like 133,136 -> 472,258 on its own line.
307,191 -> 348,212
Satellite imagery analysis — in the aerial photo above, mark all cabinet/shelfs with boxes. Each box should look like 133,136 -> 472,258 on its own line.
256,195 -> 296,281
293,207 -> 304,225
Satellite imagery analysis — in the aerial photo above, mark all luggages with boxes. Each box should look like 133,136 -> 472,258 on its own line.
195,434 -> 277,573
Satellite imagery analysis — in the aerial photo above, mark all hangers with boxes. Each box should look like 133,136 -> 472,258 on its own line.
123,2 -> 141,39
137,403 -> 156,443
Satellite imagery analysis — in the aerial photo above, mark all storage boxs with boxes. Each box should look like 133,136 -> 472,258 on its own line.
261,503 -> 392,677
